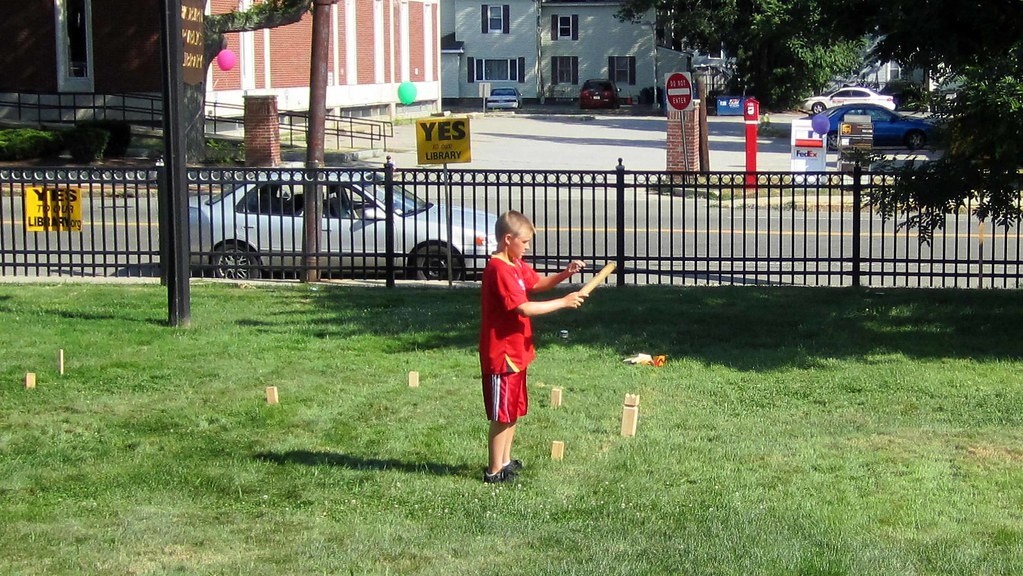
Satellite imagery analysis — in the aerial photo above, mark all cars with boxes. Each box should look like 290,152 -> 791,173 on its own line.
486,87 -> 524,110
799,86 -> 897,115
579,79 -> 623,111
800,103 -> 943,153
190,164 -> 504,283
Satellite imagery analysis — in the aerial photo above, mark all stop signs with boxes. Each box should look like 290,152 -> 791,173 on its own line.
666,73 -> 692,111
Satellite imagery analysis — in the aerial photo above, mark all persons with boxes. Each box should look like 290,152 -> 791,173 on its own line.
478,210 -> 590,483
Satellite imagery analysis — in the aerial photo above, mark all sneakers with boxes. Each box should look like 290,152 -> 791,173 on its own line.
503,459 -> 522,471
484,467 -> 518,483
